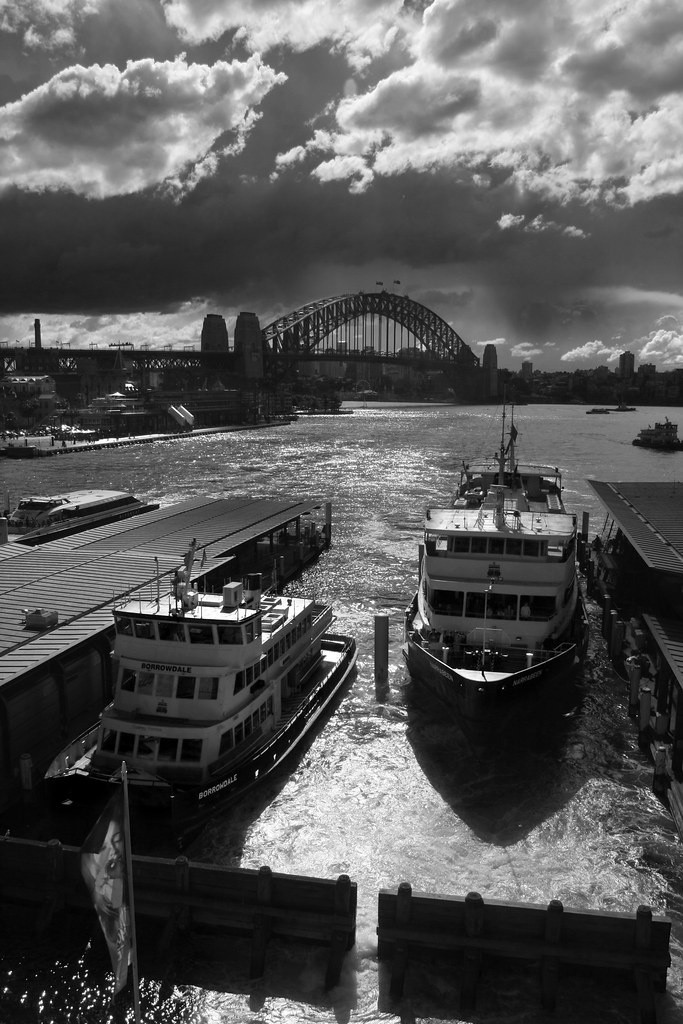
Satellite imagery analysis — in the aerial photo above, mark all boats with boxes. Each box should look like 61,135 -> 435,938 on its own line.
41,530 -> 360,855
632,415 -> 683,451
586,408 -> 609,414
4,477 -> 143,532
606,400 -> 637,411
402,384 -> 590,747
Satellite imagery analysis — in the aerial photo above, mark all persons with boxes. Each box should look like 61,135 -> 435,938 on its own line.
520,602 -> 531,617
